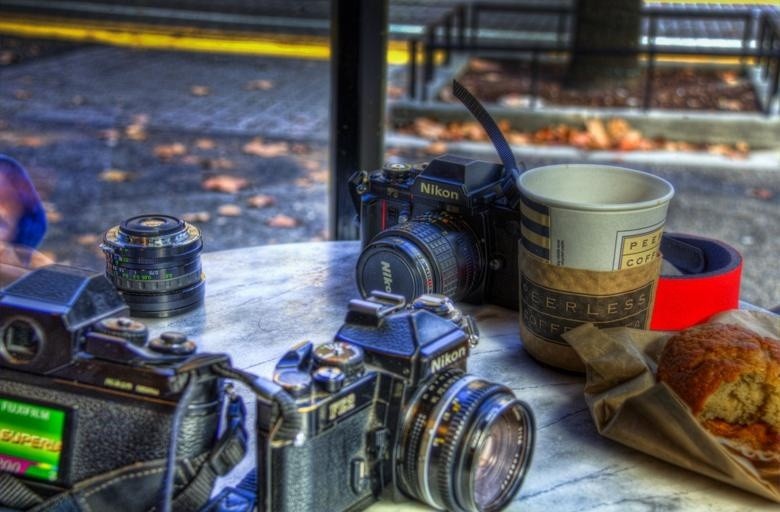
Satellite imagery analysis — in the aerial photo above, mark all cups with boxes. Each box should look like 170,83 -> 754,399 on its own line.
517,163 -> 675,373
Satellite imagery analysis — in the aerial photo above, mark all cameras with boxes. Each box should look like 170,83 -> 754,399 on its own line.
254,288 -> 537,511
0,262 -> 233,511
347,153 -> 519,315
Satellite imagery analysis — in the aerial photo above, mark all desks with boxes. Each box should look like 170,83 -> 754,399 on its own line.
109,242 -> 780,511
